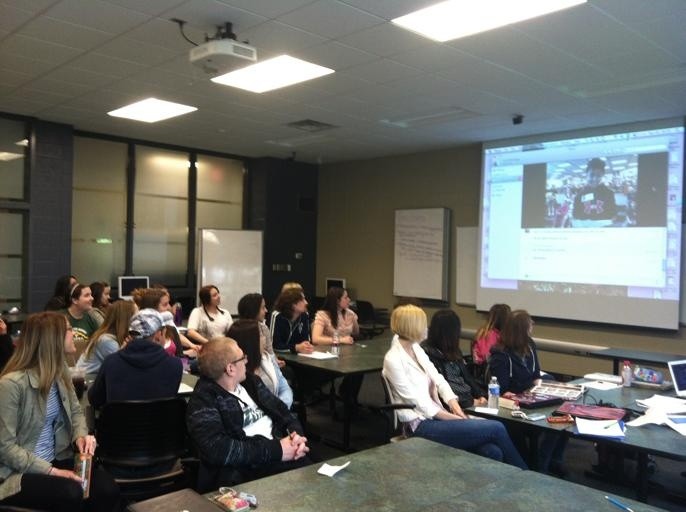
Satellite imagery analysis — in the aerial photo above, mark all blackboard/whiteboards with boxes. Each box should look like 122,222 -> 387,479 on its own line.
202,228 -> 263,316
456,222 -> 686,327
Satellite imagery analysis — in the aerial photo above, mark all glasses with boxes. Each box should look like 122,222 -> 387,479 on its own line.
224,352 -> 248,373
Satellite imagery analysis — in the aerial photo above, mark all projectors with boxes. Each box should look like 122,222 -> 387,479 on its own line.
188,38 -> 258,75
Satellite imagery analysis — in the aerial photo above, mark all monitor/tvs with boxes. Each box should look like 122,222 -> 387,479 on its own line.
325,277 -> 346,296
117,276 -> 150,301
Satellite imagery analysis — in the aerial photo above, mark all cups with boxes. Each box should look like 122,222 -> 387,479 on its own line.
69,366 -> 85,400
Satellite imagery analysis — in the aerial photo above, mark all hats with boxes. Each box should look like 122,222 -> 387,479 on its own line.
128,307 -> 174,340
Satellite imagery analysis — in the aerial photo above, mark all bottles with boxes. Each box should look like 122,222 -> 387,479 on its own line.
622,360 -> 632,387
488,377 -> 500,409
73,449 -> 92,499
331,332 -> 341,356
173,302 -> 183,326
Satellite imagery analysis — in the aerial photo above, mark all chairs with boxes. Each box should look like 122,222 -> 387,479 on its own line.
0,296 -> 686,512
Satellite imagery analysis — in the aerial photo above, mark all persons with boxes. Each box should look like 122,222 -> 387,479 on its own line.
1,275 -> 200,510
187,286 -> 233,343
570,157 -> 618,221
182,335 -> 324,489
421,309 -> 516,443
230,294 -> 294,411
484,310 -> 567,474
381,303 -> 533,471
470,303 -> 555,380
269,280 -> 364,408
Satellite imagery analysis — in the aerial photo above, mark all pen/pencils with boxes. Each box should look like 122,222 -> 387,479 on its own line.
82,459 -> 87,480
286,429 -> 292,440
604,496 -> 632,512
604,421 -> 618,429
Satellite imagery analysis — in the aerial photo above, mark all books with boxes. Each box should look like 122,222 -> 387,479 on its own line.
583,372 -> 622,383
551,402 -> 627,421
511,391 -> 562,409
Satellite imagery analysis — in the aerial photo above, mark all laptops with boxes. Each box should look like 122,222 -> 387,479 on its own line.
667,360 -> 686,398
